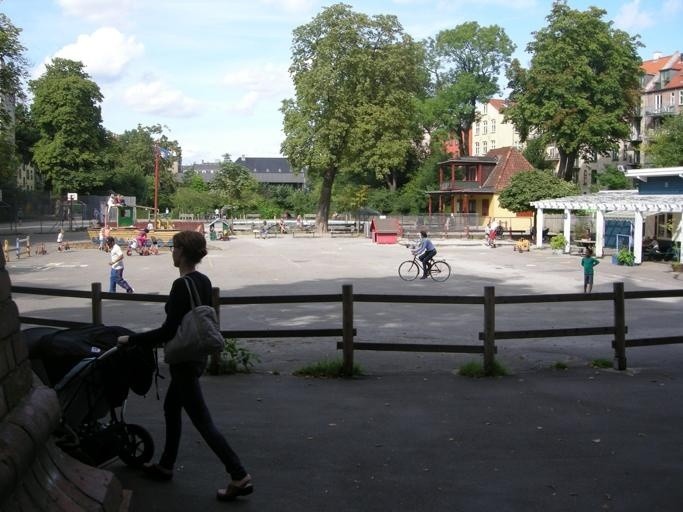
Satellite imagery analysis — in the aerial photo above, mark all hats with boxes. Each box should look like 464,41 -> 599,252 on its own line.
106,237 -> 113,244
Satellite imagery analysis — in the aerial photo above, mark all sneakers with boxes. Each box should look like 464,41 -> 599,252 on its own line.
420,275 -> 427,279
142,461 -> 173,482
217,474 -> 254,500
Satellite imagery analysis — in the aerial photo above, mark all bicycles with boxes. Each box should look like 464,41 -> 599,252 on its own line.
397,253 -> 452,283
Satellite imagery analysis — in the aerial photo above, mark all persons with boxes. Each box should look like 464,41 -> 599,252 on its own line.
485,220 -> 503,245
580,229 -> 592,249
17,208 -> 23,225
530,223 -> 536,240
57,229 -> 64,251
442,215 -> 453,236
643,235 -> 659,260
215,207 -> 226,220
412,231 -> 437,280
580,249 -> 599,293
542,228 -> 550,243
108,193 -> 126,217
106,237 -> 134,294
151,242 -> 158,249
258,212 -> 302,237
97,222 -> 112,251
115,230 -> 254,500
165,207 -> 169,218
139,229 -> 149,255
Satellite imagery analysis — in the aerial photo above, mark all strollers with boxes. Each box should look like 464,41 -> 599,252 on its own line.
18,323 -> 155,471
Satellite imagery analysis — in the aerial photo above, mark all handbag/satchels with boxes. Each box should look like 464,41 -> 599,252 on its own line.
163,306 -> 226,364
